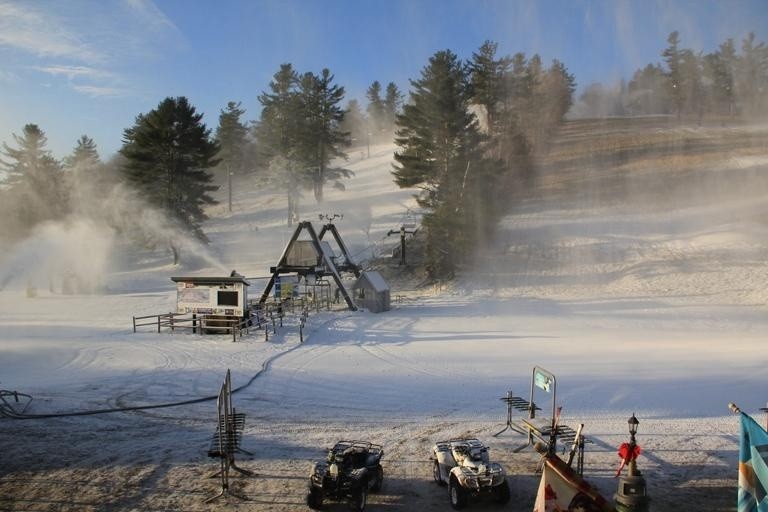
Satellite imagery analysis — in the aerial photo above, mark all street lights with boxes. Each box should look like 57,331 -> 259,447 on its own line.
625,413 -> 638,478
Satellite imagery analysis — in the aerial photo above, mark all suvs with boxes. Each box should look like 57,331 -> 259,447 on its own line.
301,439 -> 384,511
429,436 -> 510,510
